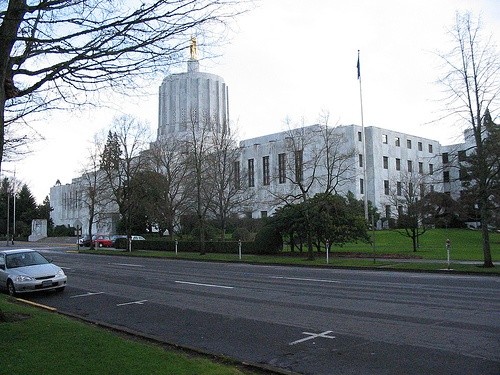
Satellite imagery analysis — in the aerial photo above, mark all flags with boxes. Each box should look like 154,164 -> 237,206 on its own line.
356,55 -> 360,79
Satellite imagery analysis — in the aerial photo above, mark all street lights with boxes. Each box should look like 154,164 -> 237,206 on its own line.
6,186 -> 11,246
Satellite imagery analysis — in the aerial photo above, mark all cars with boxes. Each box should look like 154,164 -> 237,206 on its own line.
113,236 -> 146,248
77,234 -> 96,247
91,234 -> 114,247
0,249 -> 68,298
